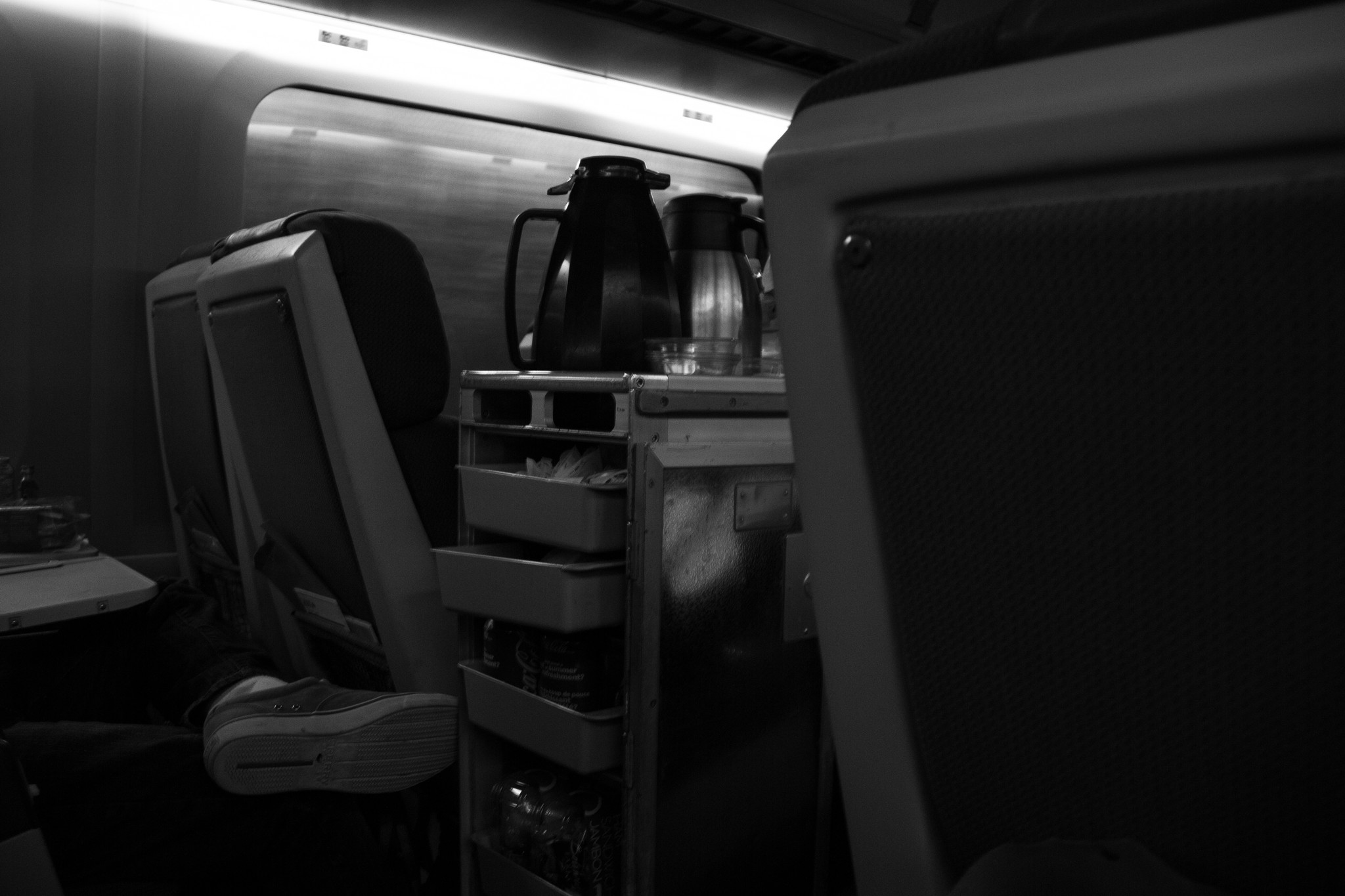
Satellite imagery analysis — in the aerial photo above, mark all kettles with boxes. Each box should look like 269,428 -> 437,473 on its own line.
654,184 -> 774,375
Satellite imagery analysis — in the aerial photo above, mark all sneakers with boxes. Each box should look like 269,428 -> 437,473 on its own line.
203,678 -> 458,795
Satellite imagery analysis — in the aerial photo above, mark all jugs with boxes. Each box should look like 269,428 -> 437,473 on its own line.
500,151 -> 682,378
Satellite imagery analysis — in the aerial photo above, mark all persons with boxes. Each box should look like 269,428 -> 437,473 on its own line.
0,574 -> 465,894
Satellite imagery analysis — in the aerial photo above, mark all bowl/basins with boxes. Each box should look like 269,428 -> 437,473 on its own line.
0,479 -> 89,552
646,332 -> 742,377
